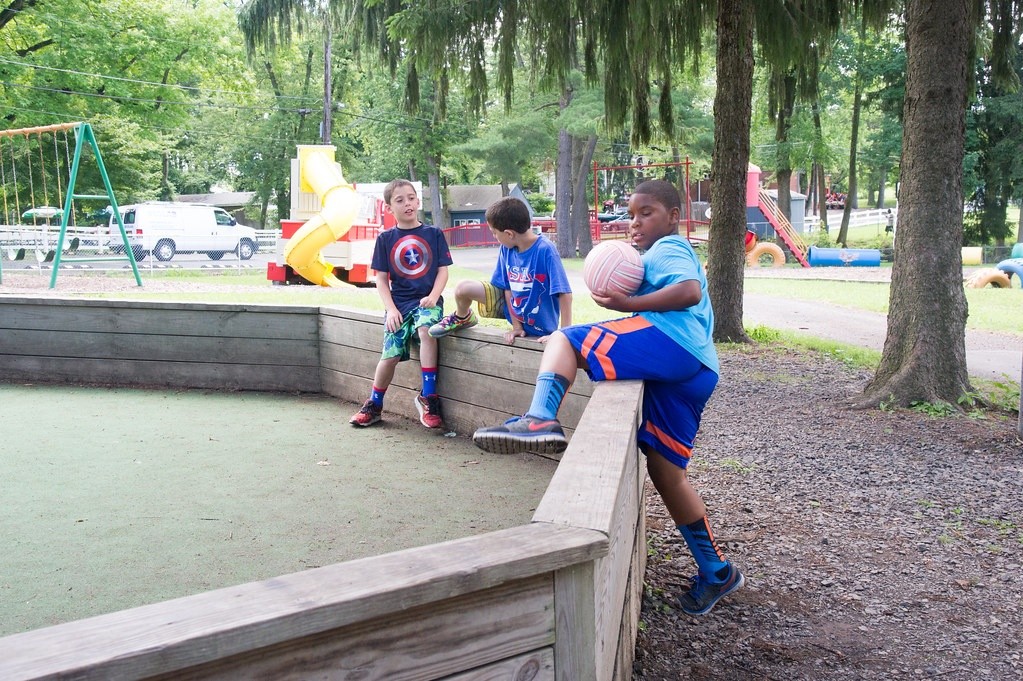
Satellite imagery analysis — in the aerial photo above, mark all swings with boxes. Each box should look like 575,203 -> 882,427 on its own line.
0,136 -> 25,261
54,132 -> 80,252
26,134 -> 55,264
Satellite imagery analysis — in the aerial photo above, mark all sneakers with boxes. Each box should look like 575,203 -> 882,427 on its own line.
472,412 -> 568,454
428,308 -> 478,338
415,388 -> 442,429
349,398 -> 382,426
675,559 -> 745,615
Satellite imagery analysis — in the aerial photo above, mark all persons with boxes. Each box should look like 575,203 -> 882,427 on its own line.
884,208 -> 894,235
472,180 -> 745,613
428,196 -> 573,346
347,179 -> 452,429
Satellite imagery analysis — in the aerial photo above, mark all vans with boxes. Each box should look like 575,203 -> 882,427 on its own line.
109,202 -> 257,261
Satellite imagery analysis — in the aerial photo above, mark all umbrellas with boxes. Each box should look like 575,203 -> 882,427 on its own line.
21,206 -> 63,219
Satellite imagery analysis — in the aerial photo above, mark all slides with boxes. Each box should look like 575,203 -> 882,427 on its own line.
282,151 -> 359,287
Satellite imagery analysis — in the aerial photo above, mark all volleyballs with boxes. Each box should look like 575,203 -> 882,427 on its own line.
581,239 -> 645,298
